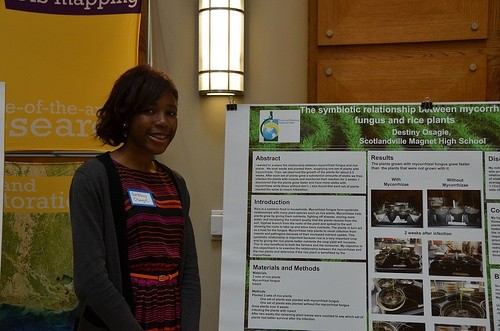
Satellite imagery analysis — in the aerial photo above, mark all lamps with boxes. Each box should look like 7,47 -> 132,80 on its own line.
197,0 -> 246,96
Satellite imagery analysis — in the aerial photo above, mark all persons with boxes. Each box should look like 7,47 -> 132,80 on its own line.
69,64 -> 202,331
